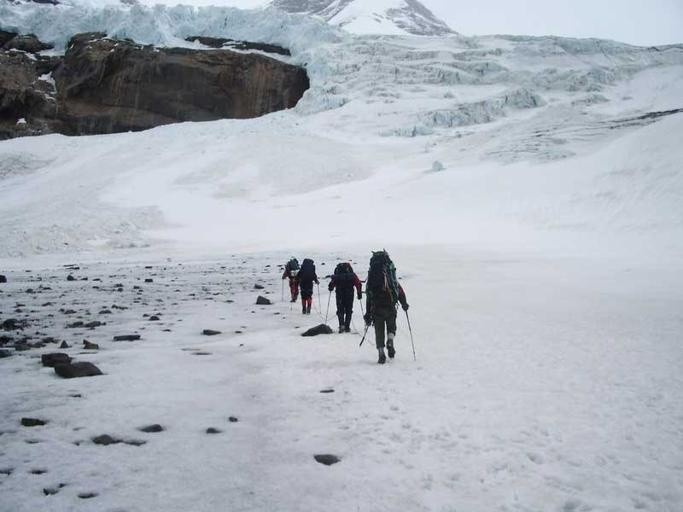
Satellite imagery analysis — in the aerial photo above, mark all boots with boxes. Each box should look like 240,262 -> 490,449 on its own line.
345,322 -> 350,332
387,340 -> 395,358
338,324 -> 346,333
378,352 -> 385,363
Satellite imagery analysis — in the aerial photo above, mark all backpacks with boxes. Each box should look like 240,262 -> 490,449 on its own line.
335,263 -> 353,289
368,251 -> 398,306
300,258 -> 314,283
287,259 -> 301,278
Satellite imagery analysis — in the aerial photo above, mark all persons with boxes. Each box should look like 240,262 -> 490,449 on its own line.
328,262 -> 362,333
364,279 -> 409,365
295,269 -> 320,316
282,258 -> 300,303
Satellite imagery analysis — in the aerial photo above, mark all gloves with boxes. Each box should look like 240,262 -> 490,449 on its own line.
328,286 -> 334,291
402,302 -> 408,310
357,293 -> 362,299
